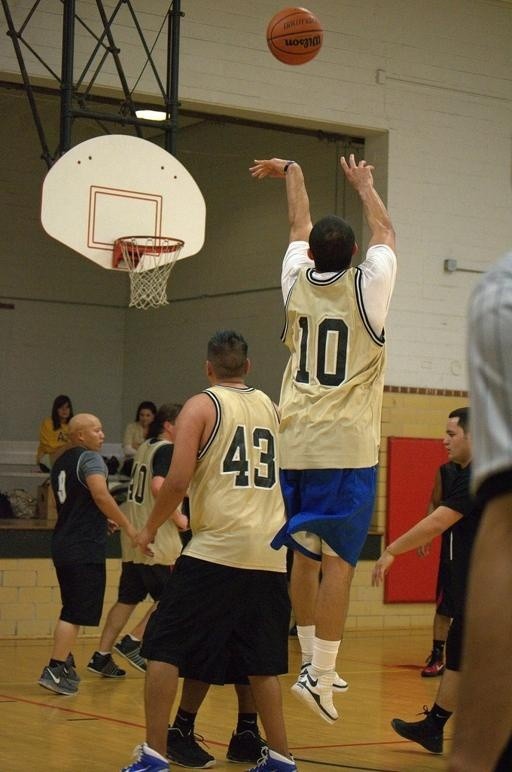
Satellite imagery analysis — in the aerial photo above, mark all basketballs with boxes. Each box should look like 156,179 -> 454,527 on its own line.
266,7 -> 324,65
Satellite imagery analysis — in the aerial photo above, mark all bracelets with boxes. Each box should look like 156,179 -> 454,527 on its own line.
386,546 -> 394,557
282,158 -> 294,174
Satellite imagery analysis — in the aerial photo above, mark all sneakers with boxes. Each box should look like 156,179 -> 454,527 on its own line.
392,718 -> 443,753
422,656 -> 444,676
119,726 -> 298,772
291,662 -> 348,724
39,632 -> 145,695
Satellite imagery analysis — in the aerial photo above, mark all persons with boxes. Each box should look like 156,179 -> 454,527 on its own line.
37,412 -> 136,695
119,401 -> 158,483
111,332 -> 297,771
371,407 -> 472,753
166,490 -> 270,769
37,394 -> 119,476
88,405 -> 189,679
446,254 -> 512,772
249,153 -> 396,723
418,459 -> 463,677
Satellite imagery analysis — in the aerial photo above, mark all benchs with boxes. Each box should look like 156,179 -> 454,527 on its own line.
2,471 -> 129,529
3,453 -> 135,476
